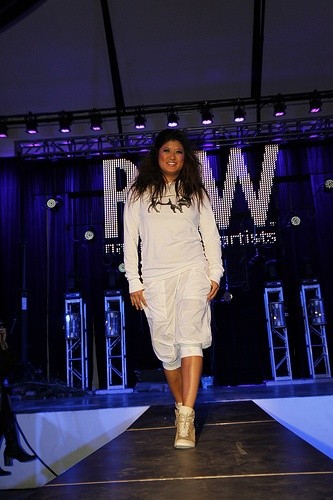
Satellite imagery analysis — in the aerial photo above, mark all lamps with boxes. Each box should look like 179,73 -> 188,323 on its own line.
0,90 -> 322,137
